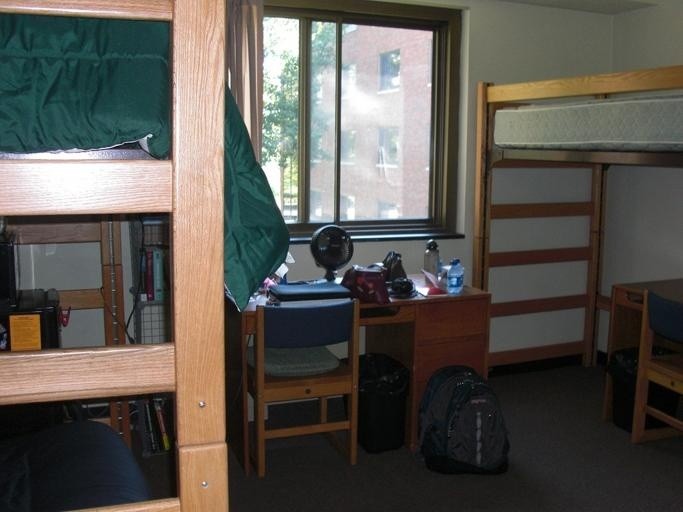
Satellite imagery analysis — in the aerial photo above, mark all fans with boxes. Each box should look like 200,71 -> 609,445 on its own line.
310,224 -> 354,282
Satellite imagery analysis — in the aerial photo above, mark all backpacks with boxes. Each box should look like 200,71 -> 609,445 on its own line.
417,365 -> 510,475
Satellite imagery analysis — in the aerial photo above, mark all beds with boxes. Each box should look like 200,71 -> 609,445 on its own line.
0,1 -> 238,511
475,65 -> 682,382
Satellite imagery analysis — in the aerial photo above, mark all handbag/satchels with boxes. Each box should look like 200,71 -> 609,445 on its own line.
341,263 -> 392,305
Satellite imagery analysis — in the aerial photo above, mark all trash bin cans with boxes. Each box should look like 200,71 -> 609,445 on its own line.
342,352 -> 411,455
610,345 -> 679,434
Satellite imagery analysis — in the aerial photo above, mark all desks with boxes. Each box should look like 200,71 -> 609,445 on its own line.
601,278 -> 683,421
224,273 -> 492,477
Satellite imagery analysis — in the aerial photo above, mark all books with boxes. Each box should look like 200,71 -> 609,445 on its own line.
146,248 -> 164,301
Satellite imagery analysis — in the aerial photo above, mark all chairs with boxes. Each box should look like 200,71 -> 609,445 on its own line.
631,286 -> 681,445
247,296 -> 361,477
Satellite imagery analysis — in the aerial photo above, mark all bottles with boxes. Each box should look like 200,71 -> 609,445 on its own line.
447,259 -> 464,297
425,240 -> 440,287
